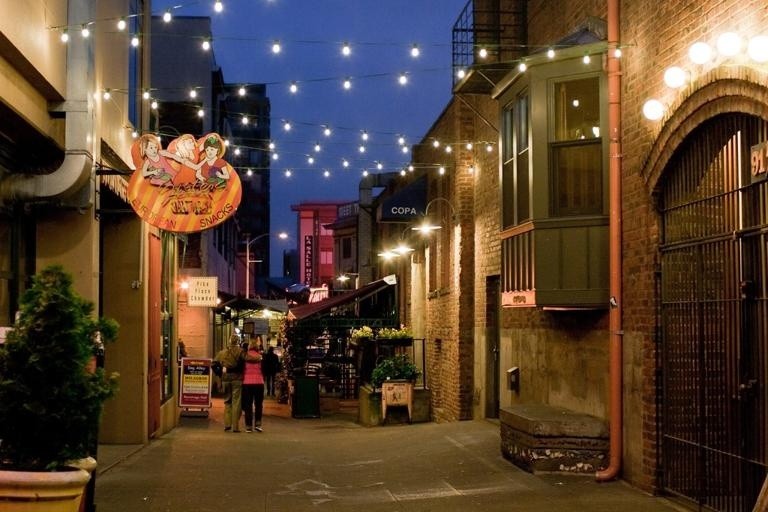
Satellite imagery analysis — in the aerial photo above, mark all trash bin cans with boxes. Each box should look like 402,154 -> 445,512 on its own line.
292,376 -> 320,418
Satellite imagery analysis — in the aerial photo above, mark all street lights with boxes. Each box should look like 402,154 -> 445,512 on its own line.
245,232 -> 288,298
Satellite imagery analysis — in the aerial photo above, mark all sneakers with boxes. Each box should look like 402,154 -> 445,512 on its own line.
224,426 -> 241,432
246,426 -> 263,433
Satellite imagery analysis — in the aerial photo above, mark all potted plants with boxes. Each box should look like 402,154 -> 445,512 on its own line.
1,262 -> 120,511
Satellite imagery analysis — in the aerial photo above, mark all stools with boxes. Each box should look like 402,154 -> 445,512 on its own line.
499,403 -> 610,475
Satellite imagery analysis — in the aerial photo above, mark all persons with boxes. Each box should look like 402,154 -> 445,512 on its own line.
243,339 -> 264,432
262,346 -> 280,397
212,334 -> 263,433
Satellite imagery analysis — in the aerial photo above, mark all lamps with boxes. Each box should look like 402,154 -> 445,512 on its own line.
593,126 -> 600,138
271,41 -> 281,53
101,79 -> 302,124
397,41 -> 623,86
50,2 -> 224,50
131,121 -> 495,178
341,41 -> 351,56
573,96 -> 579,107
343,76 -> 352,90
